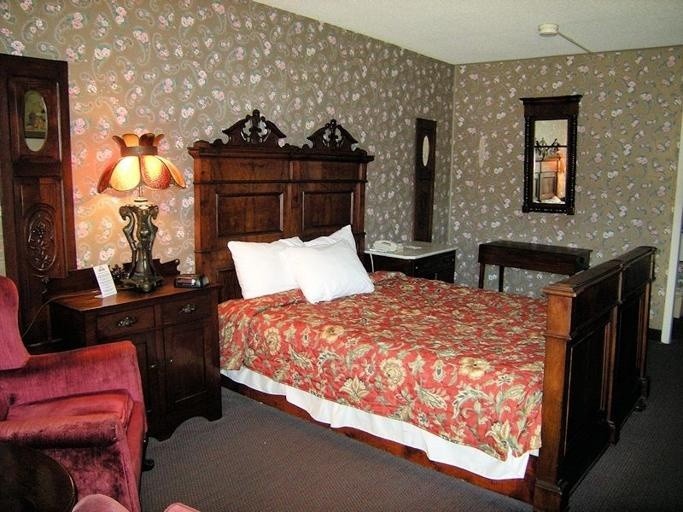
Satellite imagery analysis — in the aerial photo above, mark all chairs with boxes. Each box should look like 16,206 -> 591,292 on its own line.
72,494 -> 198,512
0,275 -> 154,512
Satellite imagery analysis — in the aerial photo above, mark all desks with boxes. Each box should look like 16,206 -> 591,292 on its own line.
0,441 -> 77,512
477,240 -> 594,292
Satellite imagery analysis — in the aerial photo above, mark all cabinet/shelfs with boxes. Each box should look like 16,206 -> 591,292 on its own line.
46,259 -> 223,441
534,158 -> 560,202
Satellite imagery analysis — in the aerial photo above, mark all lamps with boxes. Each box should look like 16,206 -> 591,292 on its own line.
97,133 -> 186,294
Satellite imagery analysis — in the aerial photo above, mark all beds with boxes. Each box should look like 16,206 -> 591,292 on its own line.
189,109 -> 658,512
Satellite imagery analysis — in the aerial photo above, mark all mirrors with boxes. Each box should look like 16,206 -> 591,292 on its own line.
518,95 -> 582,215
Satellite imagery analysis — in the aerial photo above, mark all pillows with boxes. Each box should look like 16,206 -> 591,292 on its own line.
305,224 -> 358,253
227,236 -> 302,300
285,240 -> 375,304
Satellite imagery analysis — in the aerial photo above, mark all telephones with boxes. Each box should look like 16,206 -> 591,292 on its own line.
373,240 -> 404,253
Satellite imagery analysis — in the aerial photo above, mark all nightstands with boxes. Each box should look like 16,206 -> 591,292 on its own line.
364,240 -> 458,284
